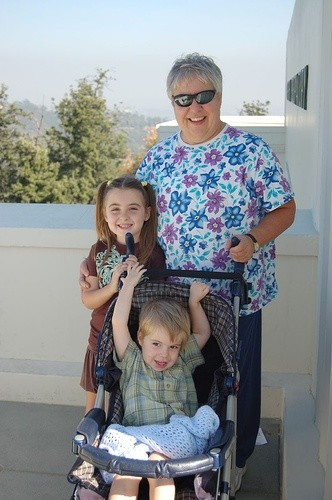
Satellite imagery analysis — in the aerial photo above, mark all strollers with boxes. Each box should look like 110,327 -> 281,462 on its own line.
70,237 -> 246,500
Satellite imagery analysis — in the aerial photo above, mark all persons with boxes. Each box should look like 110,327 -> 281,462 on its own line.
80,52 -> 296,496
79,179 -> 166,413
109,258 -> 211,500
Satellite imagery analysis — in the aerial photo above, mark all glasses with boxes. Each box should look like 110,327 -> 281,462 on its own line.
172,90 -> 217,108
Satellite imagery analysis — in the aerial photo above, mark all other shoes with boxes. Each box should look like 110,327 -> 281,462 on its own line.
234,464 -> 247,492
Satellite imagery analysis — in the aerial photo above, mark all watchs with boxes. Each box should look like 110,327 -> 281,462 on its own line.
245,234 -> 260,254
